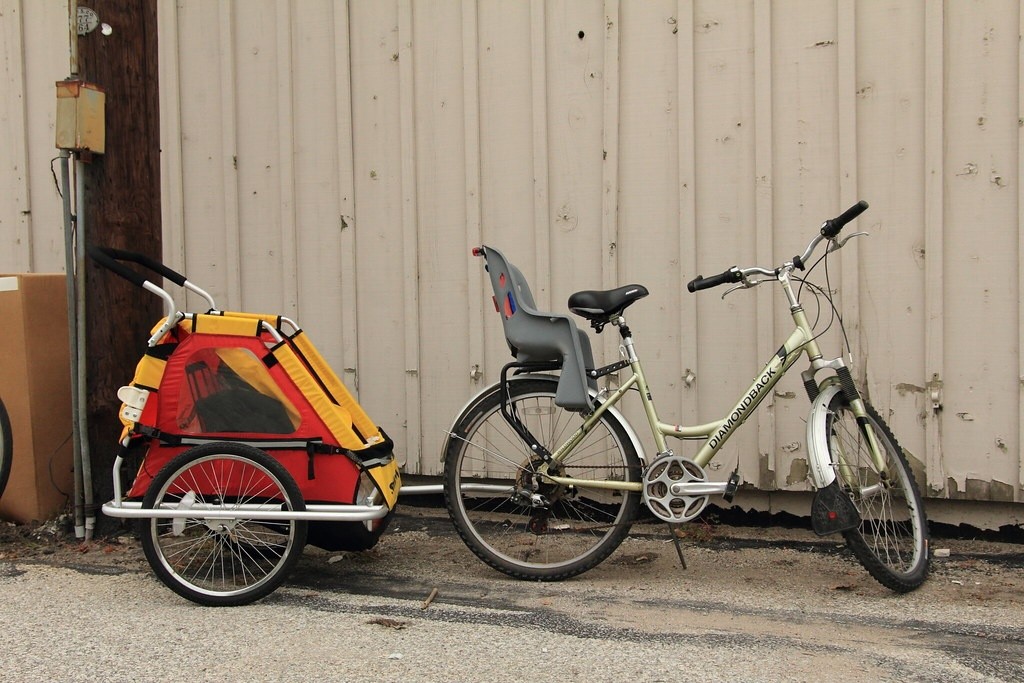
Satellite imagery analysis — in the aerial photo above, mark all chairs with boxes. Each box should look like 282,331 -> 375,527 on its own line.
472,244 -> 600,412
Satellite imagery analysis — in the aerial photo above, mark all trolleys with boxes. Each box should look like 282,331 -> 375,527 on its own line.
81,242 -> 408,608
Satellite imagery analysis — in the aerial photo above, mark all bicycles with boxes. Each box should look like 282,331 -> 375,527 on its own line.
440,198 -> 930,593
